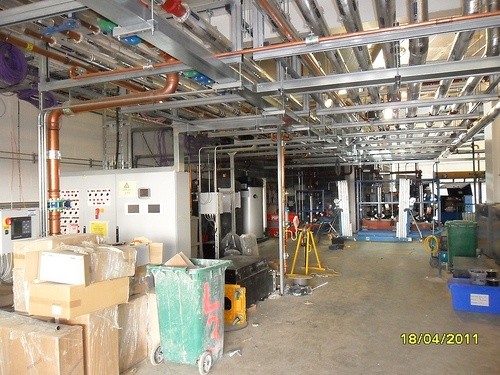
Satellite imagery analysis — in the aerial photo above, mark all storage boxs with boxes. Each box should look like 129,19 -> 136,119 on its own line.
0,233 -> 155,375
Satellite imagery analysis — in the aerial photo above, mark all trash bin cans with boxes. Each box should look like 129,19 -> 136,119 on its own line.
445,220 -> 480,271
146,257 -> 234,375
